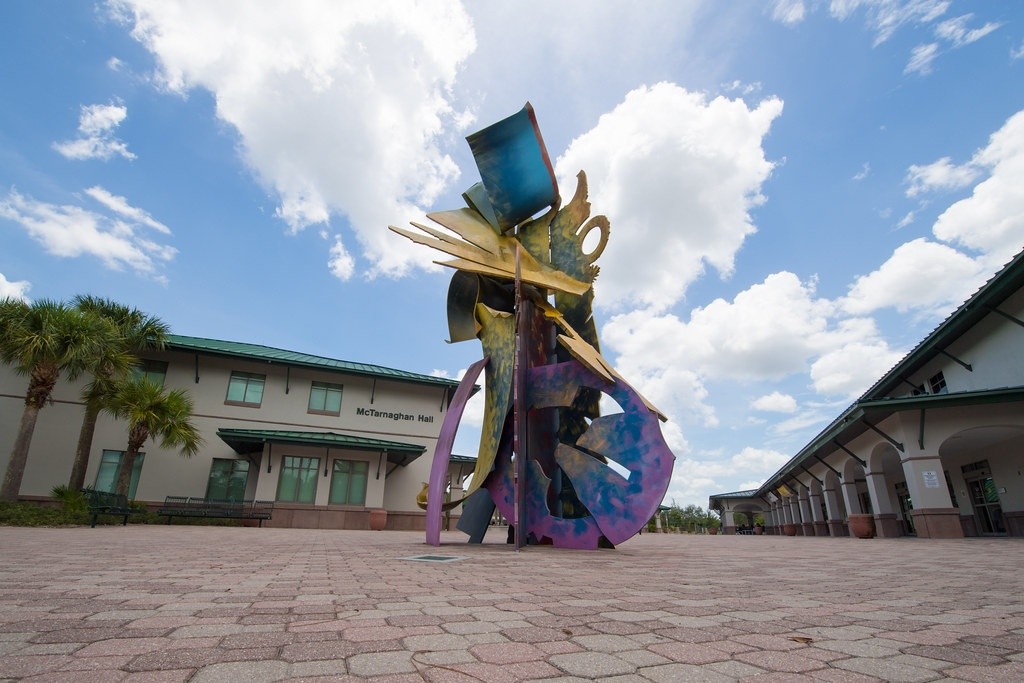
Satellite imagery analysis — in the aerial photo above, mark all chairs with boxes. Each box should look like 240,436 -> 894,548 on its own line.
159,495 -> 275,529
80,489 -> 142,529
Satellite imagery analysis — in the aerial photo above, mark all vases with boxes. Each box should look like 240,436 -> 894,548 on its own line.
784,524 -> 796,536
848,514 -> 876,540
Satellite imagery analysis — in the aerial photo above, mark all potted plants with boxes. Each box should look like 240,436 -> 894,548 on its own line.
706,517 -> 721,535
753,514 -> 766,535
